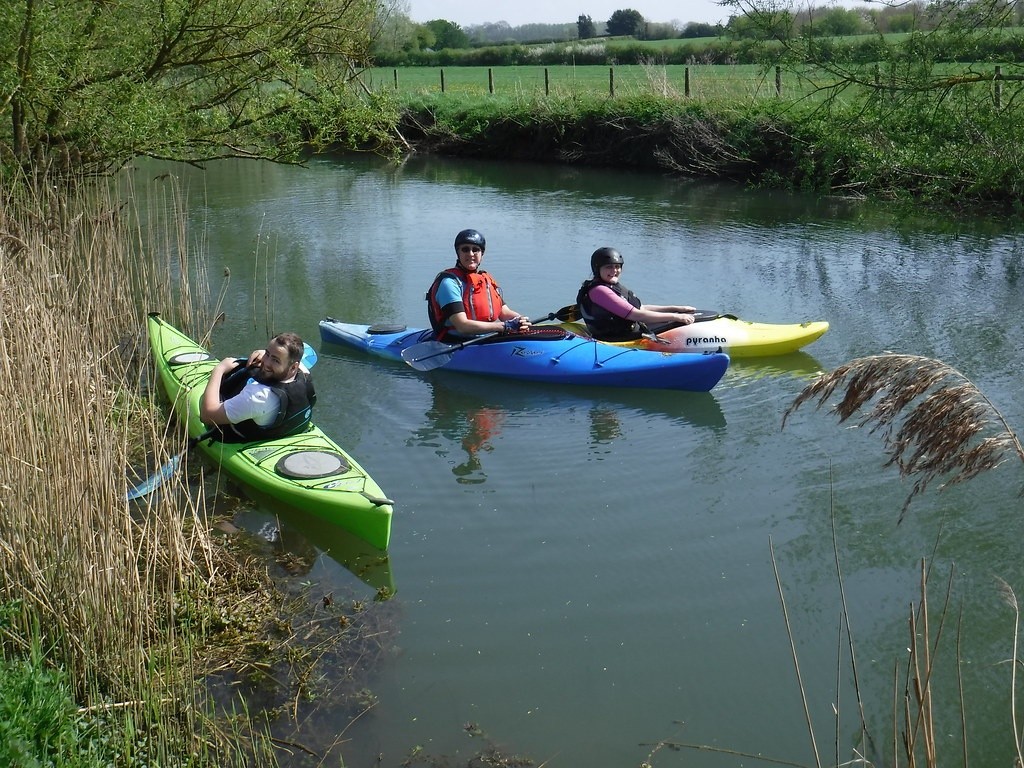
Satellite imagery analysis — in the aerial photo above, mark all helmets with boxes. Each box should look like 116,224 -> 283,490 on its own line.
455,229 -> 485,253
591,247 -> 624,275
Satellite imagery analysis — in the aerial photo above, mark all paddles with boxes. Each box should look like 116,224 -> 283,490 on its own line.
120,339 -> 320,502
399,303 -> 583,373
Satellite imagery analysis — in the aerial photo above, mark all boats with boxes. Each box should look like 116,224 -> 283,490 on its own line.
317,320 -> 729,393
235,483 -> 396,597
557,309 -> 830,358
147,312 -> 395,550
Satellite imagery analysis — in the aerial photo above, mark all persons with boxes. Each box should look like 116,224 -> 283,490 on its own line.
577,247 -> 696,342
200,332 -> 317,443
425,229 -> 532,345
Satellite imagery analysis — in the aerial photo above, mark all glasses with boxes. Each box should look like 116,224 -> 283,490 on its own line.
458,246 -> 482,253
